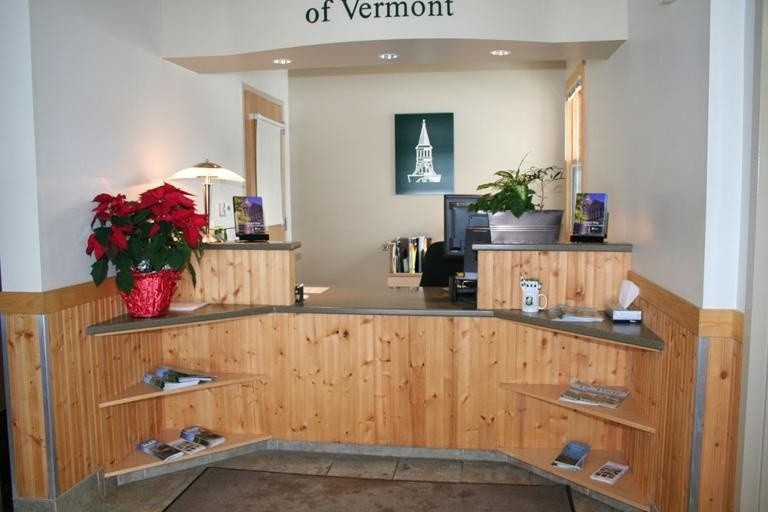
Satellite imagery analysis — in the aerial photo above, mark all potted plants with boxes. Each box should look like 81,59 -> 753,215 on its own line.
467,152 -> 566,245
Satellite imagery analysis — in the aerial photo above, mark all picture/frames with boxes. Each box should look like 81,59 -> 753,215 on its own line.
233,196 -> 269,241
570,193 -> 609,243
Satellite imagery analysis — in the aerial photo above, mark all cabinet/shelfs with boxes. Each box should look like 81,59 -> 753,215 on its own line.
493,307 -> 663,511
87,305 -> 272,495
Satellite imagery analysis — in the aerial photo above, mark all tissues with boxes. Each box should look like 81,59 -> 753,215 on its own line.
606,279 -> 643,321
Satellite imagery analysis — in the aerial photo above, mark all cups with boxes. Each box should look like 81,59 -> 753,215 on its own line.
520,280 -> 548,313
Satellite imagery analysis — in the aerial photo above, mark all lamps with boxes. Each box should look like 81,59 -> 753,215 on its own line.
166,158 -> 245,243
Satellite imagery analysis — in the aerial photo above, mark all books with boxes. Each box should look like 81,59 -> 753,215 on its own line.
552,439 -> 592,471
549,306 -> 595,322
572,192 -> 608,237
575,383 -> 631,410
142,363 -> 217,390
558,379 -> 600,405
138,425 -> 226,462
555,303 -> 605,321
590,460 -> 632,485
390,235 -> 427,274
232,196 -> 265,234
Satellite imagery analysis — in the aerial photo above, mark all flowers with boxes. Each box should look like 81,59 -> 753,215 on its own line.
86,182 -> 208,296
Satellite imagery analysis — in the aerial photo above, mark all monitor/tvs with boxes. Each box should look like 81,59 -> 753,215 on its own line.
444,194 -> 489,280
